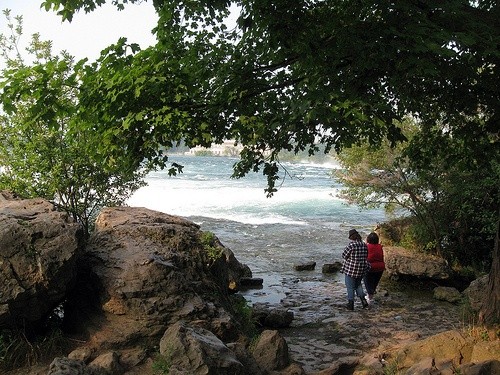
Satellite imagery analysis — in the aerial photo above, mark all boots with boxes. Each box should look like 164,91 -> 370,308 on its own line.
361,298 -> 369,308
345,300 -> 354,311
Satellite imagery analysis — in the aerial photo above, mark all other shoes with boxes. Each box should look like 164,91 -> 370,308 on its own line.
365,295 -> 374,301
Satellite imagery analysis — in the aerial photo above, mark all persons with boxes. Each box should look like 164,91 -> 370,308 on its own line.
341,229 -> 371,311
362,232 -> 386,297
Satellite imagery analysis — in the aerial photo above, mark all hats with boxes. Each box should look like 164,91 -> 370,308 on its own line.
349,229 -> 358,238
